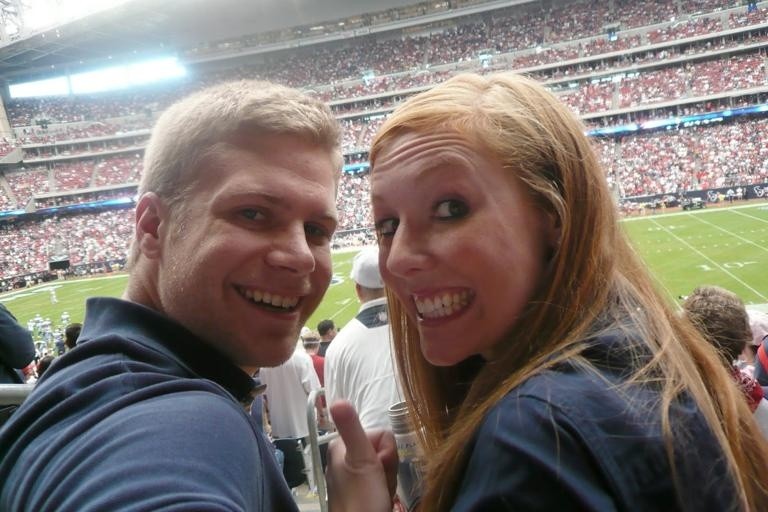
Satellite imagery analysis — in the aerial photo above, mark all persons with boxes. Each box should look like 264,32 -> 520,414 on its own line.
1,0 -> 768,512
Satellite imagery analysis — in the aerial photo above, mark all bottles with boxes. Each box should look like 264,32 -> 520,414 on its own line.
386,399 -> 430,511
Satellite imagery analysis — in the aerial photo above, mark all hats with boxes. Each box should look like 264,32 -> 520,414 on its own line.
300,325 -> 319,343
350,246 -> 385,289
748,320 -> 768,347
